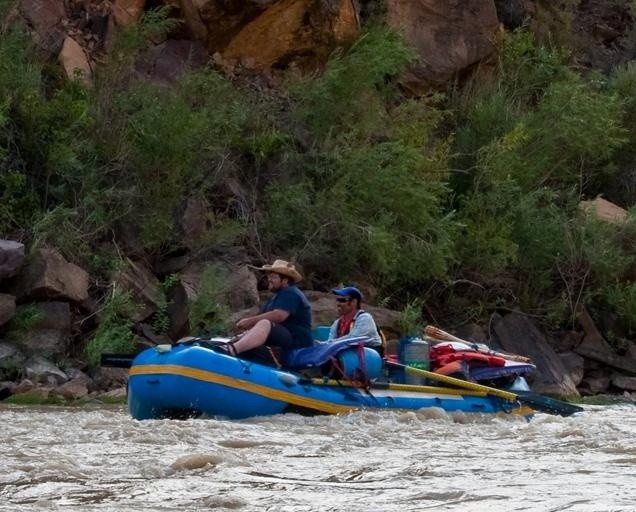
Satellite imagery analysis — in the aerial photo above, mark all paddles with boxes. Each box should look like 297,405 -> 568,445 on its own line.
101,353 -> 137,367
370,380 -> 541,409
386,361 -> 583,417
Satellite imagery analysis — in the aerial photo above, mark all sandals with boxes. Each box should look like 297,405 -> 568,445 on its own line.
214,345 -> 237,357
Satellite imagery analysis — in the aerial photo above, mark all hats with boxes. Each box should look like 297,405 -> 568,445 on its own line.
263,259 -> 303,283
332,286 -> 362,300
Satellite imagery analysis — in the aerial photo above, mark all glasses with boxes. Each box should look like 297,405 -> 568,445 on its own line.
336,298 -> 352,302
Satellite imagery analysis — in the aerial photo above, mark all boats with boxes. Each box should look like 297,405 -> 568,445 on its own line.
128,345 -> 534,420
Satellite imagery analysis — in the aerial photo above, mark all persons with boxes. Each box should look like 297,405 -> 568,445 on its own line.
313,286 -> 384,356
214,260 -> 312,368
